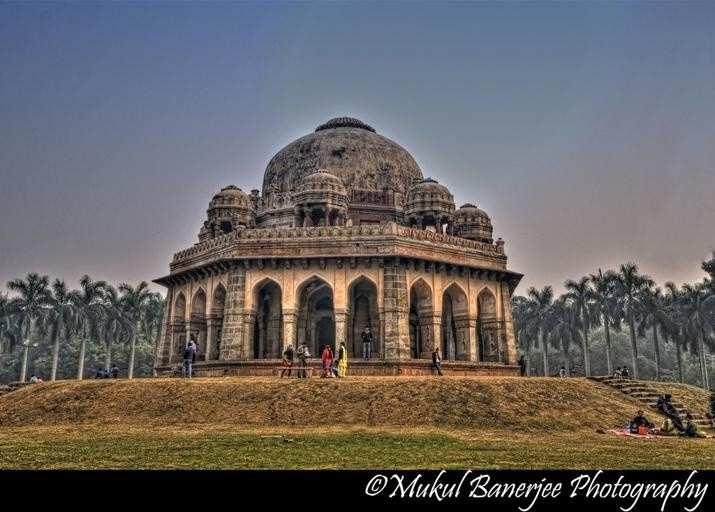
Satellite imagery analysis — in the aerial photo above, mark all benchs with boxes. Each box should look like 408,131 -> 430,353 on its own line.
278,367 -> 314,380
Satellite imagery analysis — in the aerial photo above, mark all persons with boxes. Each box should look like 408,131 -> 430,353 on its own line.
524,360 -> 529,377
30,374 -> 38,383
95,368 -> 104,378
104,370 -> 110,378
518,355 -> 525,377
622,366 -> 629,376
613,367 -> 621,377
630,392 -> 698,437
559,367 -> 567,378
111,364 -> 120,378
178,333 -> 198,380
570,366 -> 576,375
37,377 -> 43,383
361,325 -> 373,361
280,341 -> 347,378
432,348 -> 444,377
